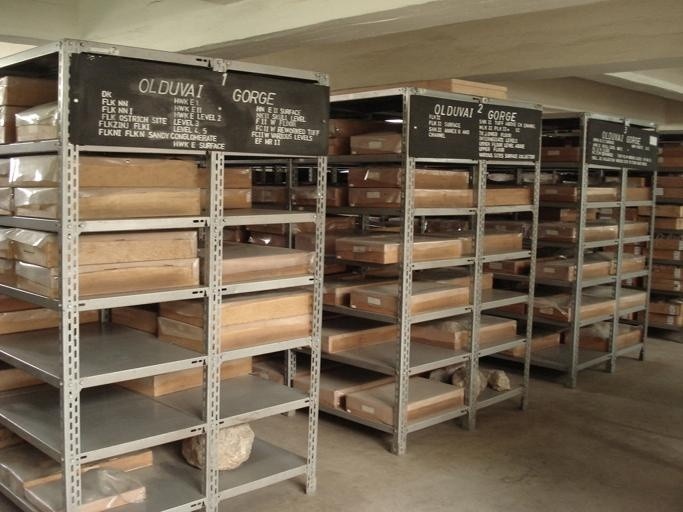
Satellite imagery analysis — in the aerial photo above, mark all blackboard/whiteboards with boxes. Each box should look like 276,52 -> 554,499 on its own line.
71,52 -> 330,157
585,118 -> 661,172
408,95 -> 542,164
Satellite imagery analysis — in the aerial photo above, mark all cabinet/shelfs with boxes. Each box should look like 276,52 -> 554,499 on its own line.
317,111 -> 683,388
2,39 -> 330,512
199,86 -> 546,458
